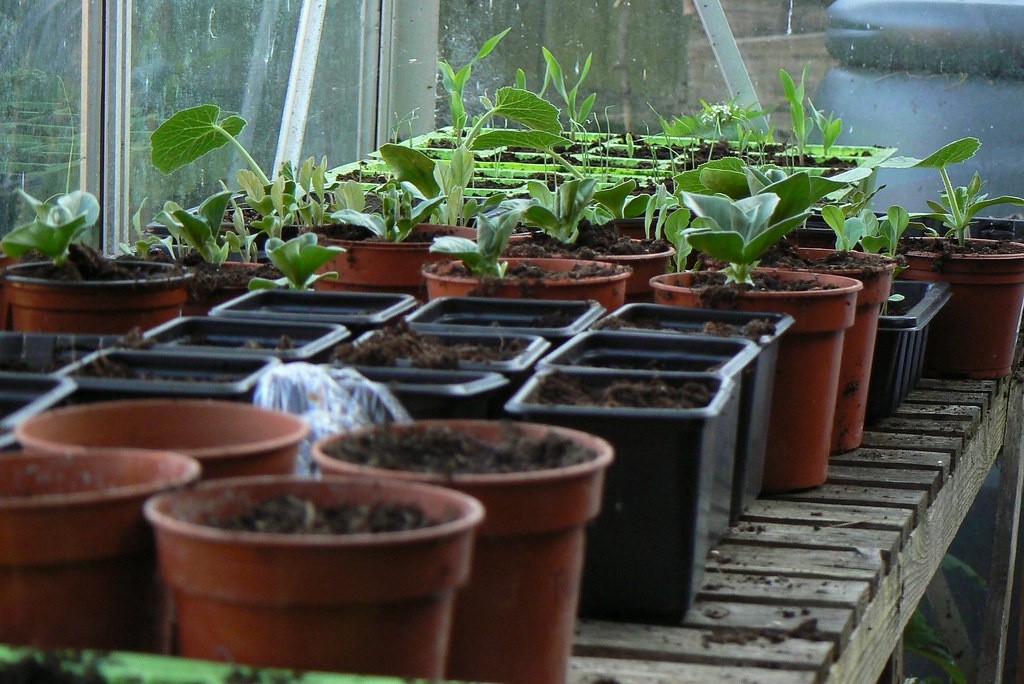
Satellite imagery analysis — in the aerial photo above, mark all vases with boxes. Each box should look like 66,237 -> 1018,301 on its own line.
505,368 -> 732,628
144,317 -> 346,363
144,477 -> 483,680
406,297 -> 603,339
209,288 -> 416,326
0,450 -> 199,651
58,347 -> 282,402
341,332 -> 546,370
16,399 -> 308,473
589,303 -> 798,517
536,332 -> 758,551
312,419 -> 612,684
357,373 -> 511,415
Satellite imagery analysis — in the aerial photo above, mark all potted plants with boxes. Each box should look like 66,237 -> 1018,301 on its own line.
887,139 -> 1024,378
500,179 -> 676,305
282,28 -> 893,203
649,190 -> 862,492
302,189 -> 479,304
130,202 -> 258,299
822,201 -> 949,415
701,165 -> 881,452
421,207 -> 631,311
4,188 -> 187,332
